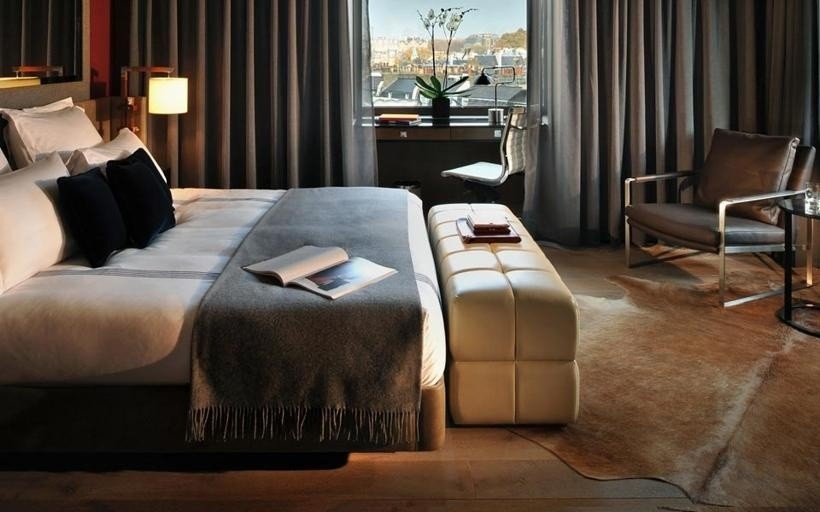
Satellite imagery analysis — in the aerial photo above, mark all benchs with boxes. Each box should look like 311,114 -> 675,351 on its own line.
427,203 -> 581,428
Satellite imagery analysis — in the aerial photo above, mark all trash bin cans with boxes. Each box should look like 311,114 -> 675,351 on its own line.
392,180 -> 424,200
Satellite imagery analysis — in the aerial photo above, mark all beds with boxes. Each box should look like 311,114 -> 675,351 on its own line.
0,100 -> 450,452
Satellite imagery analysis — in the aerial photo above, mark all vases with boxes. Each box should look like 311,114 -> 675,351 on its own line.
432,98 -> 450,124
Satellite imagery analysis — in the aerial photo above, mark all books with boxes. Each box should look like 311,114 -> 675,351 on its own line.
456,212 -> 521,244
0,76 -> 40,89
241,244 -> 399,300
374,113 -> 422,126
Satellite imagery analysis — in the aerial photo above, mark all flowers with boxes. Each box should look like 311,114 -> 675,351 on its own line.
411,5 -> 485,108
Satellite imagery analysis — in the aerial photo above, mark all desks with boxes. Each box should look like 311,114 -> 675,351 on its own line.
775,196 -> 819,339
351,112 -> 509,144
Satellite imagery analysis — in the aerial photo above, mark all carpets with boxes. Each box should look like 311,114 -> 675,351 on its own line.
499,244 -> 820,512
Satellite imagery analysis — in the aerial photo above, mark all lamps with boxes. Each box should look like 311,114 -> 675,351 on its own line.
475,65 -> 516,124
117,65 -> 190,135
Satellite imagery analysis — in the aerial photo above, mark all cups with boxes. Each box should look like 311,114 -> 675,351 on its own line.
804,181 -> 820,214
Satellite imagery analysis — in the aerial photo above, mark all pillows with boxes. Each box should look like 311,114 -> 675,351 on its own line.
694,127 -> 801,226
0,94 -> 185,294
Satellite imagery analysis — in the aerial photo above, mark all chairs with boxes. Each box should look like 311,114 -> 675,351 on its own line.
439,102 -> 543,202
620,130 -> 816,309
